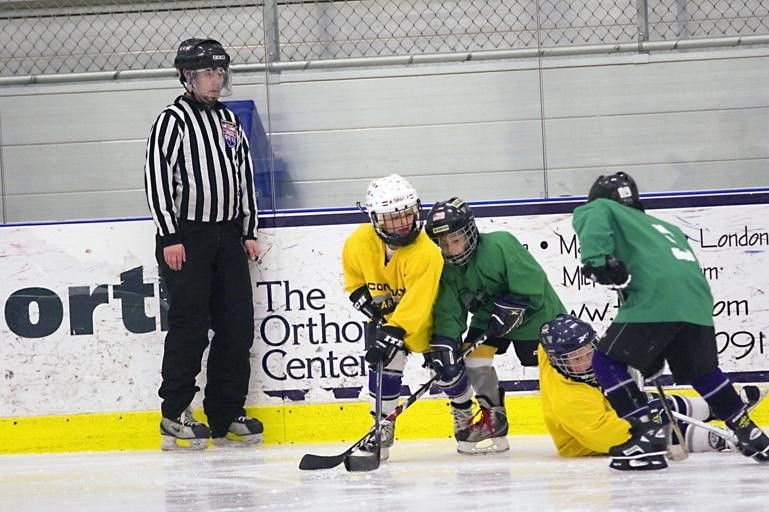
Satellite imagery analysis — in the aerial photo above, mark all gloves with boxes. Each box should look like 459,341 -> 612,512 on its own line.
366,327 -> 403,369
584,263 -> 633,291
348,287 -> 387,328
485,293 -> 527,341
426,335 -> 462,383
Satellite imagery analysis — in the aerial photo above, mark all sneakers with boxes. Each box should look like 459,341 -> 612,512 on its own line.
727,410 -> 769,460
449,401 -> 475,443
732,380 -> 769,408
214,413 -> 264,436
458,391 -> 510,442
608,457 -> 669,471
347,413 -> 395,461
607,423 -> 667,458
161,410 -> 211,441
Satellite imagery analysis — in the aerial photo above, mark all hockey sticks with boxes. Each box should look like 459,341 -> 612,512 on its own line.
346,314 -> 381,470
612,286 -> 691,461
300,329 -> 489,470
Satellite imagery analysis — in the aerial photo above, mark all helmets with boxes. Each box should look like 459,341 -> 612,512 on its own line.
540,315 -> 604,384
174,38 -> 234,97
358,176 -> 425,244
590,172 -> 642,209
425,198 -> 480,266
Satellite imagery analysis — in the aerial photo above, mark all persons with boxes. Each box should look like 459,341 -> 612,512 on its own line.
573,172 -> 769,470
538,315 -> 763,458
144,40 -> 264,450
425,198 -> 568,455
343,177 -> 472,462
